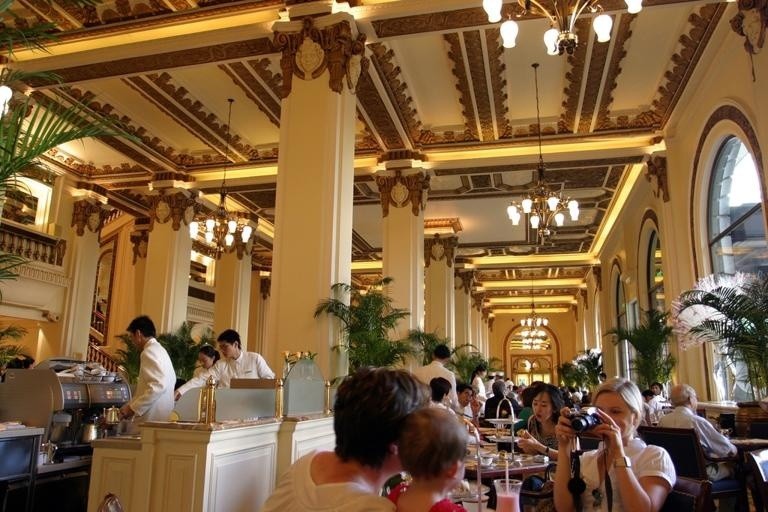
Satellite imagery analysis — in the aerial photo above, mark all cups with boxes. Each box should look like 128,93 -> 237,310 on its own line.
481,456 -> 494,466
461,495 -> 490,512
493,478 -> 524,512
720,428 -> 730,440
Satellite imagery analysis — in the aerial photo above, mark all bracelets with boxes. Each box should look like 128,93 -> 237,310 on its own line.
545,445 -> 549,456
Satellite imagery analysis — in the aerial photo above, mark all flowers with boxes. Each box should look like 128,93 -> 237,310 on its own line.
283,351 -> 318,365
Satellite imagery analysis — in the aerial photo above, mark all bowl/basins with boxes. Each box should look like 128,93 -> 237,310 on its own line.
71,363 -> 117,383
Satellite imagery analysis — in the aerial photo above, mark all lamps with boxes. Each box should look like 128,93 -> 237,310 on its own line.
520,273 -> 548,349
507,64 -> 579,236
483,0 -> 642,55
189,100 -> 258,251
0,68 -> 12,116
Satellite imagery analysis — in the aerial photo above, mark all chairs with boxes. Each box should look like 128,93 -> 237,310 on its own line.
579,432 -> 601,450
520,463 -> 558,512
636,426 -> 749,512
747,453 -> 768,512
662,476 -> 712,512
97,493 -> 124,512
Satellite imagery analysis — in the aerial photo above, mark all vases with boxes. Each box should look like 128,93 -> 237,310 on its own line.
283,363 -> 293,379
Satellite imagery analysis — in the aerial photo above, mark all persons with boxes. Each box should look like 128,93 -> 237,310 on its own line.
514,384 -> 583,482
413,333 -> 605,420
552,376 -> 677,512
256,366 -> 432,512
387,409 -> 471,512
114,313 -> 276,434
639,380 -> 740,480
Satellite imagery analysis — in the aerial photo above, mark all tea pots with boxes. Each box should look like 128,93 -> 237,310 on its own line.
102,404 -> 121,426
79,415 -> 99,443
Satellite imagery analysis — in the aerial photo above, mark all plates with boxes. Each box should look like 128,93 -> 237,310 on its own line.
481,463 -> 497,468
485,434 -> 523,442
488,453 -> 524,462
485,417 -> 523,424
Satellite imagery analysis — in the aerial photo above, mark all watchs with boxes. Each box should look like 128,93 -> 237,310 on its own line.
612,456 -> 632,468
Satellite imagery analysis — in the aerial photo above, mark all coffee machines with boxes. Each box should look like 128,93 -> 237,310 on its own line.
0,370 -> 133,472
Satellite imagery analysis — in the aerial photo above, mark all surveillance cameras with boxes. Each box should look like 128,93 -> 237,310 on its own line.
49,312 -> 61,322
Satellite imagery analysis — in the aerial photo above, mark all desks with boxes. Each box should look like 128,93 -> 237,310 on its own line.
697,399 -> 768,438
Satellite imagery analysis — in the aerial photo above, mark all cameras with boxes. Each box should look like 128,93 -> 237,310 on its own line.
567,412 -> 602,432
533,455 -> 549,463
